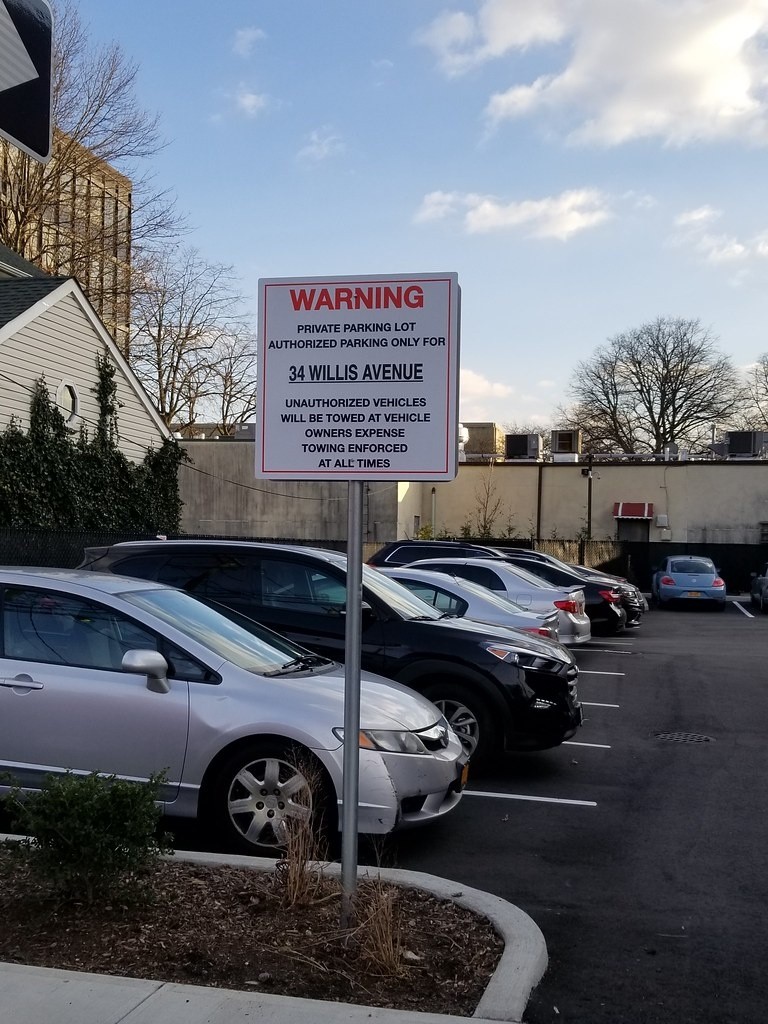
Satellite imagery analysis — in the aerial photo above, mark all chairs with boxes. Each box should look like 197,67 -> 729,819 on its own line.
73,605 -> 124,670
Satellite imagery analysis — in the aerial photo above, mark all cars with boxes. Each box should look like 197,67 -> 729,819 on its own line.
273,565 -> 560,643
501,557 -> 624,637
489,545 -> 649,629
397,557 -> 593,646
1,567 -> 470,855
749,562 -> 768,615
652,554 -> 727,612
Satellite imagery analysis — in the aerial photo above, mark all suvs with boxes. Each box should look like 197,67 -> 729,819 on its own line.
66,535 -> 586,774
361,538 -> 508,563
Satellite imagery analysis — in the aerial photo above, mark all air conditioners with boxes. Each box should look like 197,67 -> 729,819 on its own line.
506,434 -> 543,459
551,429 -> 582,454
